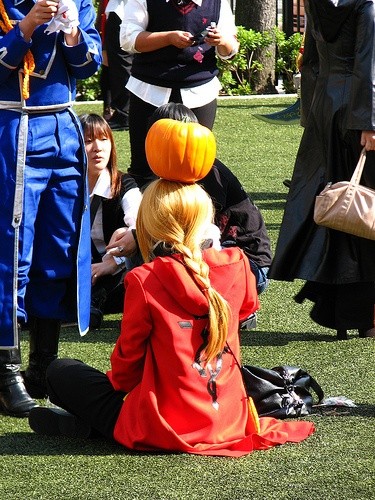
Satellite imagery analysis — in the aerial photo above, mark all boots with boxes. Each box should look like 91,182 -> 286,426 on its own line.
0,348 -> 38,417
25,314 -> 60,397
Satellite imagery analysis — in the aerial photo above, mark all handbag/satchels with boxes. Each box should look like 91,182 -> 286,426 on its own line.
241,365 -> 325,417
314,148 -> 375,239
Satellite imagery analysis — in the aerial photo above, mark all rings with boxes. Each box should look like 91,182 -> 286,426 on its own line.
94,274 -> 98,279
117,247 -> 124,254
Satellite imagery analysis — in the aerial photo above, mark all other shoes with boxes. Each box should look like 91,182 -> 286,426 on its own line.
337,329 -> 349,340
103,106 -> 114,121
88,288 -> 107,317
29,406 -> 76,435
107,120 -> 130,131
241,312 -> 258,328
283,180 -> 292,187
359,325 -> 375,338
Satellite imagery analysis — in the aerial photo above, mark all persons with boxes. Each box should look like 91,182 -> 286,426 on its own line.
267,0 -> 375,342
0,0 -> 375,342
0,0 -> 103,417
77,113 -> 144,334
103,103 -> 273,329
28,178 -> 315,458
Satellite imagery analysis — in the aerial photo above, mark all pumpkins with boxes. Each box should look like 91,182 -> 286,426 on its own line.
144,116 -> 216,183
